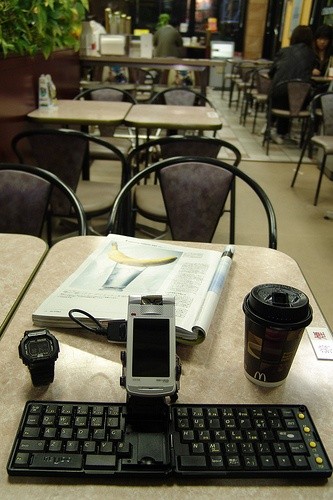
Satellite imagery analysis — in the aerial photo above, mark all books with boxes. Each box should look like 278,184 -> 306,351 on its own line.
32,232 -> 234,345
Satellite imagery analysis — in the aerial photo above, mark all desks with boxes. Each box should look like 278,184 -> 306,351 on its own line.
0,231 -> 333,500
311,75 -> 333,82
26,98 -> 132,181
123,103 -> 223,186
78,53 -> 227,105
0,232 -> 49,341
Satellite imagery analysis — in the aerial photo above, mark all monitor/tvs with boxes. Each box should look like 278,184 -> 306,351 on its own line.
210,40 -> 235,59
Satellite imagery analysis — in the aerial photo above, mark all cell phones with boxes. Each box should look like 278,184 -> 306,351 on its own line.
127,294 -> 178,395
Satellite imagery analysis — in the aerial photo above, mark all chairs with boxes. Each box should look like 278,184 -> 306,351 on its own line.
0,50 -> 333,250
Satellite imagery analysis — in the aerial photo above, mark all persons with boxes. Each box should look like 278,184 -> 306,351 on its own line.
151,20 -> 183,58
260,23 -> 333,145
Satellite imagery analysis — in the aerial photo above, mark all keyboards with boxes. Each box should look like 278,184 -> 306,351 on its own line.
6,401 -> 333,480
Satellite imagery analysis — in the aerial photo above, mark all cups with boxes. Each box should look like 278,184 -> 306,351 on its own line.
180,23 -> 189,33
208,17 -> 217,32
242,284 -> 314,387
182,36 -> 198,46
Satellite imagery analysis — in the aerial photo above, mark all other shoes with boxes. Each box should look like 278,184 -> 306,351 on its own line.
260,122 -> 291,144
